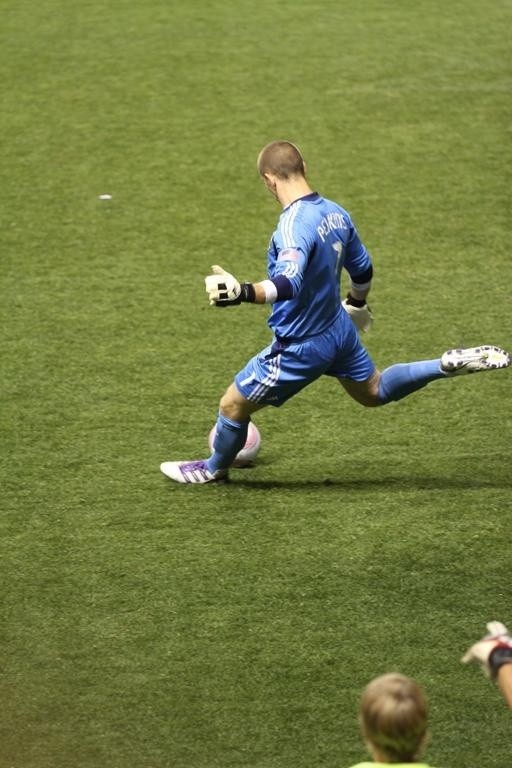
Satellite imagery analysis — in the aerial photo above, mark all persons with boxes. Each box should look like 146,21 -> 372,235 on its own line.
460,620 -> 512,710
349,671 -> 434,768
158,139 -> 512,486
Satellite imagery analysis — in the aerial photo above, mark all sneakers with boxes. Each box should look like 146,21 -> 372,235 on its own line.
160,461 -> 227,484
440,345 -> 510,375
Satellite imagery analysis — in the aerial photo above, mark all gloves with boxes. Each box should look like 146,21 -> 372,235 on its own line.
342,297 -> 374,333
462,622 -> 512,679
205,265 -> 256,307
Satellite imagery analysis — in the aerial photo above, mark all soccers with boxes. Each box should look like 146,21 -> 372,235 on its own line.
208,414 -> 261,468
